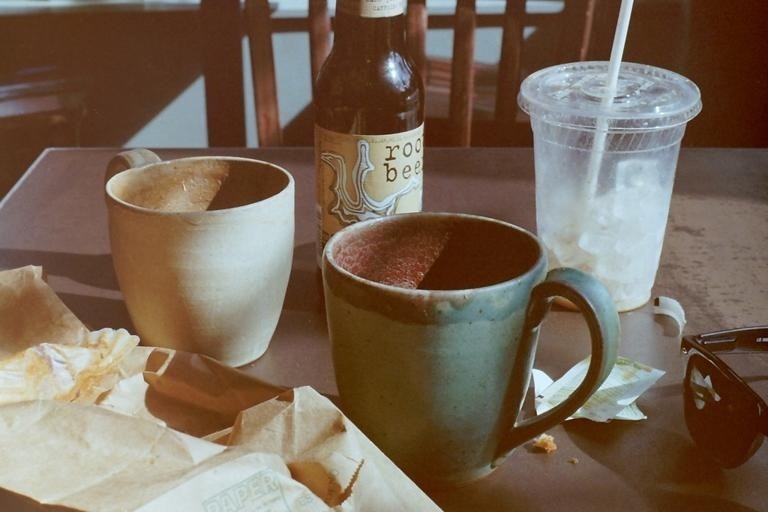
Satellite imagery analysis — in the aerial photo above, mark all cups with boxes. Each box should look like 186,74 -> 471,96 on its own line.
513,59 -> 705,313
322,211 -> 621,493
99,148 -> 299,367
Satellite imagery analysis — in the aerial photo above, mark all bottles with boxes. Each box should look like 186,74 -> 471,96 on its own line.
314,0 -> 424,273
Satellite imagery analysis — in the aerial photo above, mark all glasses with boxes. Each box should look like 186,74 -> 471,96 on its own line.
680,326 -> 768,471
